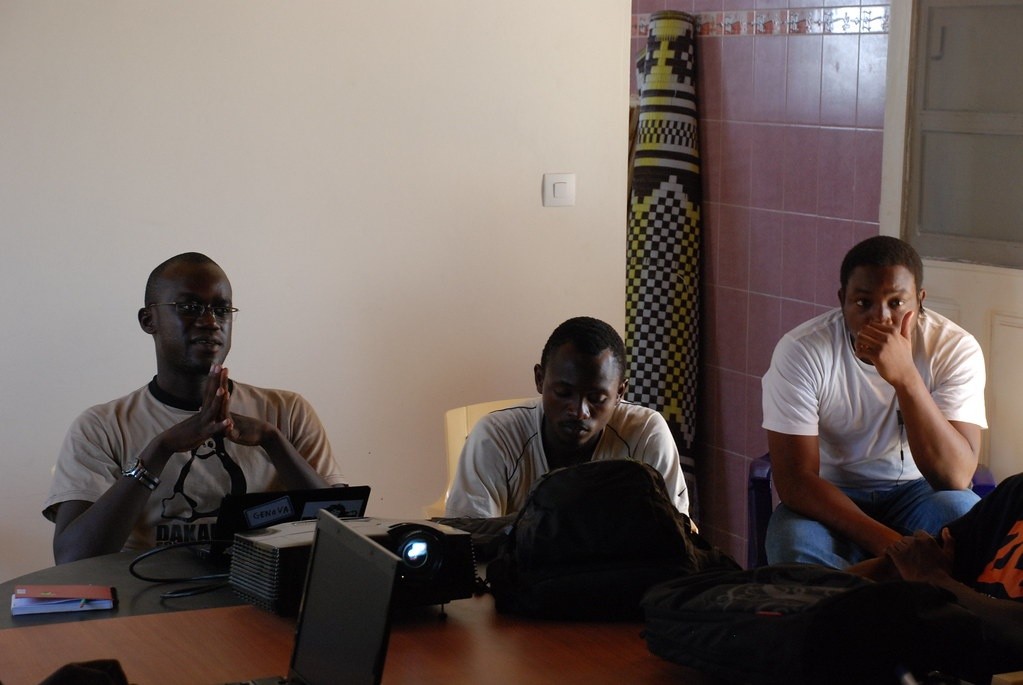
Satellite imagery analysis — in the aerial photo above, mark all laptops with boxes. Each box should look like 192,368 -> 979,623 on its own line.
185,484 -> 372,569
222,508 -> 403,685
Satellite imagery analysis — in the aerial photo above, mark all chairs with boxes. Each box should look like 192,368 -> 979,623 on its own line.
420,396 -> 541,521
746,452 -> 996,581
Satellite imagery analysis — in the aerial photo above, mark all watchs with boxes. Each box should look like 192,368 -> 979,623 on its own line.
121,457 -> 161,489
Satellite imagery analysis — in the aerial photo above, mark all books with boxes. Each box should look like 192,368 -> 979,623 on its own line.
11,585 -> 119,615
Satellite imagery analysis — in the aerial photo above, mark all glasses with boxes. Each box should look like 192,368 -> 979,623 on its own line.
149,300 -> 239,323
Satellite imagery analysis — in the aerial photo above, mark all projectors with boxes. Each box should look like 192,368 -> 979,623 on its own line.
228,514 -> 478,618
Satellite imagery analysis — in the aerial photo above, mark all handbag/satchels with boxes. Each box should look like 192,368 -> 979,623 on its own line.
486,457 -> 745,622
637,560 -> 994,685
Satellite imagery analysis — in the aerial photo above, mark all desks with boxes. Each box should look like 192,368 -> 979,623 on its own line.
0,518 -> 666,685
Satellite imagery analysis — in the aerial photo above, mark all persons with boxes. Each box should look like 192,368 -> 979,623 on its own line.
445,317 -> 698,534
762,236 -> 1023,685
42,252 -> 343,565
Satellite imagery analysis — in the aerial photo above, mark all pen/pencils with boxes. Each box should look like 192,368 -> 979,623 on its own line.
79,583 -> 93,608
